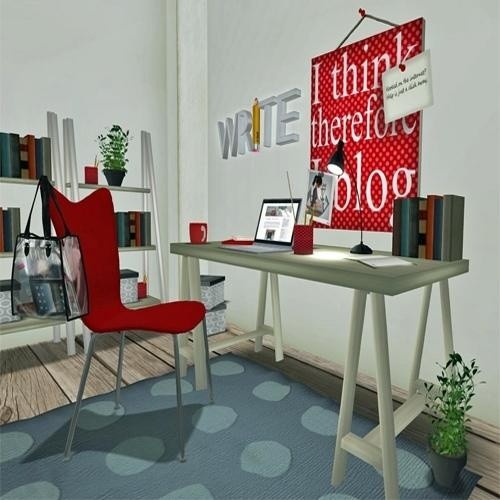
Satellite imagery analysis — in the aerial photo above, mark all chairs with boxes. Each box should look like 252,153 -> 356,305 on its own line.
34,179 -> 215,463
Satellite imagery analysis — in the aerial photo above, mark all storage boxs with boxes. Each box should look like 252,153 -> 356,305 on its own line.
119,268 -> 140,305
203,303 -> 228,337
199,273 -> 226,310
1,279 -> 24,325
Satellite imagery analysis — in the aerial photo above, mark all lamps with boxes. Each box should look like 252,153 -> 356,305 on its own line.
327,139 -> 373,253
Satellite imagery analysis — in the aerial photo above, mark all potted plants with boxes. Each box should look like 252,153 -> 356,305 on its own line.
93,123 -> 131,186
417,351 -> 488,489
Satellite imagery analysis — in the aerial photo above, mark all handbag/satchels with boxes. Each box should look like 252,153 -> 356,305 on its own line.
10,233 -> 89,321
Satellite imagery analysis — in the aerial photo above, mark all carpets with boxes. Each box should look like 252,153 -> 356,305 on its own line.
0,351 -> 483,498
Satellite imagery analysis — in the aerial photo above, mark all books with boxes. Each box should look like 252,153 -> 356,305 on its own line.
0,132 -> 51,252
392,194 -> 464,262
115,211 -> 151,247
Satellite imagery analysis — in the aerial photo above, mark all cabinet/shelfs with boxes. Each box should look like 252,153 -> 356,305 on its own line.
61,119 -> 167,354
1,109 -> 77,356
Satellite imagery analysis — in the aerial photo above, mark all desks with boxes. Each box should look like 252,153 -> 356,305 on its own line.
169,236 -> 468,498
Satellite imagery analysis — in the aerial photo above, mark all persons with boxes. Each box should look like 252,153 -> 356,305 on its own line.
308,175 -> 325,216
